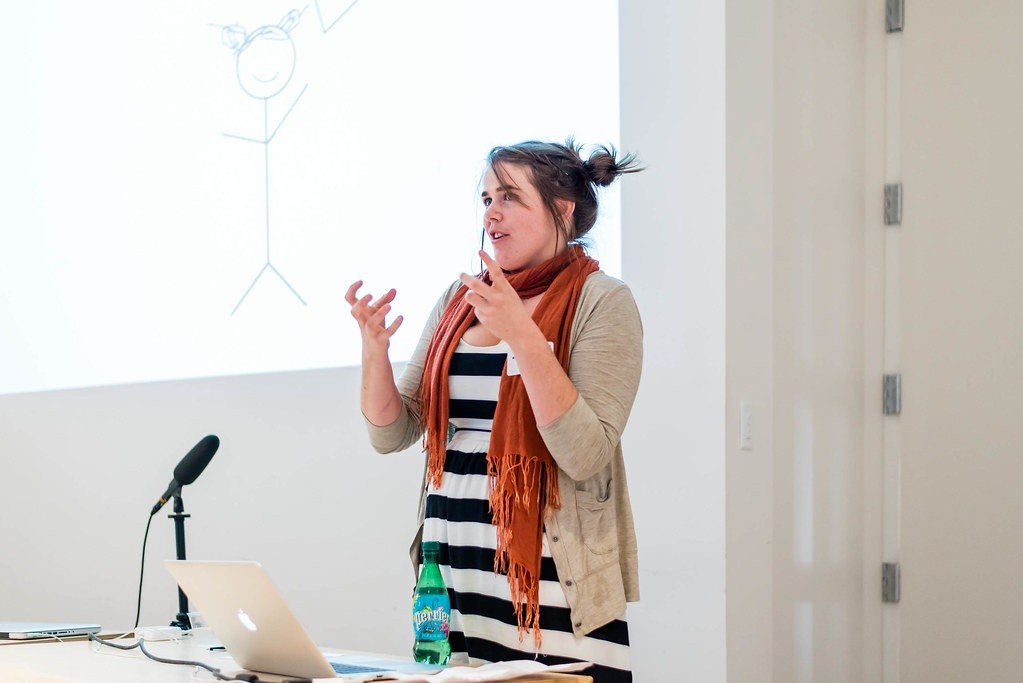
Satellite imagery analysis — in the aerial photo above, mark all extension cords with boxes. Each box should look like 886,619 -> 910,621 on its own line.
135,625 -> 181,641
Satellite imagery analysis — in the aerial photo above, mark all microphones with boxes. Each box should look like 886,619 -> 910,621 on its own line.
151,435 -> 219,515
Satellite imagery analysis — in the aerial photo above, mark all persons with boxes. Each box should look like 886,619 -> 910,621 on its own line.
345,140 -> 643,682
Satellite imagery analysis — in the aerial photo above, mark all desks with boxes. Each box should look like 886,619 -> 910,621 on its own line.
0,631 -> 598,683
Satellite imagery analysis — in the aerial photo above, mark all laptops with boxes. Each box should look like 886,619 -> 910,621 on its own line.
0,622 -> 101,639
164,559 -> 444,681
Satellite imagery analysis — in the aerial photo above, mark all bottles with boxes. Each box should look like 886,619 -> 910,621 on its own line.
413,542 -> 452,665
